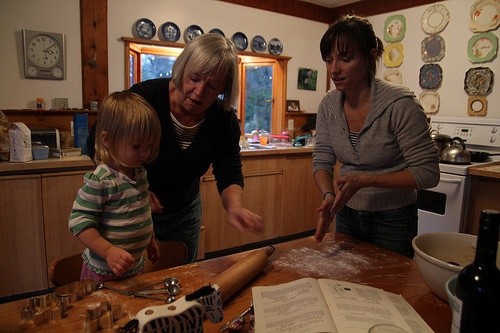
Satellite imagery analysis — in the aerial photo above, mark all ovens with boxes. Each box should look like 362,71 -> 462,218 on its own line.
414,162 -> 472,235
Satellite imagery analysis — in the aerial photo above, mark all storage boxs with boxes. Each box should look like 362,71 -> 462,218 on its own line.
9,122 -> 32,162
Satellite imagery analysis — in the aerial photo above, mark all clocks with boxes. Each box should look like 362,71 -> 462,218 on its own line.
22,30 -> 66,80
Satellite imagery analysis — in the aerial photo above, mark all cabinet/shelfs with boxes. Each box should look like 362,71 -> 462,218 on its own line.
0,147 -> 343,298
463,162 -> 500,241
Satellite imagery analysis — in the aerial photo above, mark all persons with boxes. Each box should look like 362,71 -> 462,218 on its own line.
311,16 -> 440,257
88,34 -> 264,266
68,93 -> 161,282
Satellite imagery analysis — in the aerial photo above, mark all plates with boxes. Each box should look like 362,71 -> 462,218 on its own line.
184,25 -> 205,44
135,18 -> 157,40
383,0 -> 500,116
268,38 -> 283,55
209,28 -> 225,38
162,22 -> 181,42
232,32 -> 248,50
252,35 -> 267,54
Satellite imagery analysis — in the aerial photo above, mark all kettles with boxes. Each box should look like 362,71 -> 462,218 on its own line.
440,136 -> 471,165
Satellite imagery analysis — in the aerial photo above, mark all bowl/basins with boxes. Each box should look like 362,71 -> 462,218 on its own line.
412,232 -> 500,305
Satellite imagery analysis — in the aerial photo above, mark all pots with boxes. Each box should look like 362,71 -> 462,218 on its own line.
469,151 -> 490,162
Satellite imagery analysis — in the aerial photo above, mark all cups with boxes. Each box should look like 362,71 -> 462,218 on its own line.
259,133 -> 268,146
444,275 -> 460,309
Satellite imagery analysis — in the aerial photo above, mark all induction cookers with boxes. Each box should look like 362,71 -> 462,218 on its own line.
429,117 -> 500,165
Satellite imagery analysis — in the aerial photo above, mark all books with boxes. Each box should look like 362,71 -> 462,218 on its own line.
252,278 -> 434,333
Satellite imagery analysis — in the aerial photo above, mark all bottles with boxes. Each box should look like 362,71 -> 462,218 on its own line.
451,210 -> 500,333
269,135 -> 281,145
280,131 -> 290,146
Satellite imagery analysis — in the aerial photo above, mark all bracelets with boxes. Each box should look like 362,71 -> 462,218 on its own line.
324,192 -> 335,200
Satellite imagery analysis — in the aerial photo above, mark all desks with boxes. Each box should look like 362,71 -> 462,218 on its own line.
0,231 -> 453,333
